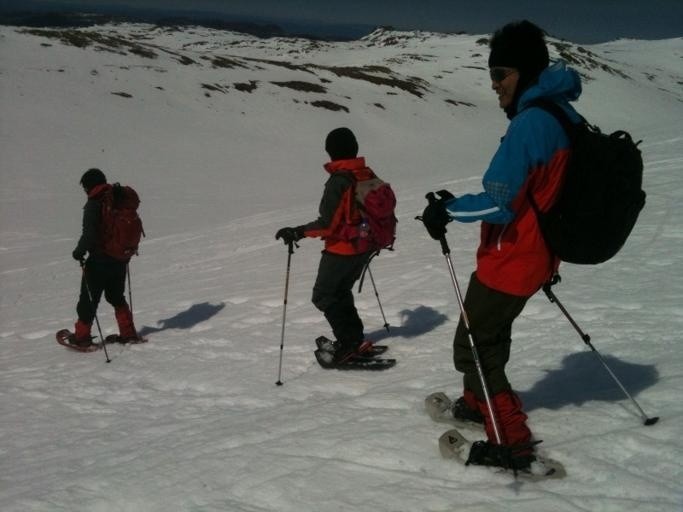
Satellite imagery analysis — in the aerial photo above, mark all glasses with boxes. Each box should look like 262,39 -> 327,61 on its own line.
488,67 -> 514,83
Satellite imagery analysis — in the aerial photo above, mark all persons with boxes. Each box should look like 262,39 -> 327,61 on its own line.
68,167 -> 136,348
276,126 -> 374,366
423,18 -> 587,470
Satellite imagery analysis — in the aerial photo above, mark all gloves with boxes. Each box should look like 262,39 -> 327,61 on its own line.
72,240 -> 89,260
422,199 -> 451,239
275,227 -> 297,245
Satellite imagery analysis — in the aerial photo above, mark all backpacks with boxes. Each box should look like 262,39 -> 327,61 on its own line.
102,183 -> 146,262
343,168 -> 398,254
522,99 -> 646,266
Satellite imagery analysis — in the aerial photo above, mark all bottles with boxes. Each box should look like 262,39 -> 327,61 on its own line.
357,217 -> 371,254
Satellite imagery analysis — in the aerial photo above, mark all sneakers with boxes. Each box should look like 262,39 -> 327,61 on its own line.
450,396 -> 484,423
115,335 -> 138,343
69,332 -> 93,348
459,440 -> 536,469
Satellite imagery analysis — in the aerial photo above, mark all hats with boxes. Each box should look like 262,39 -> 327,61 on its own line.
81,168 -> 108,195
324,127 -> 358,160
488,20 -> 549,73
